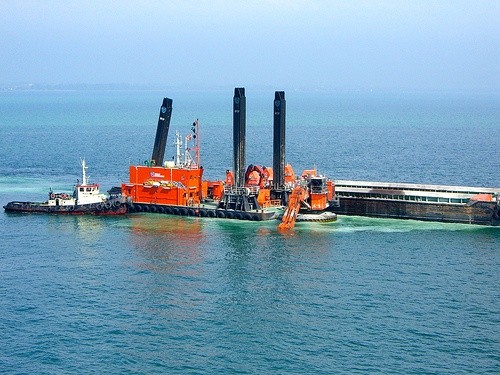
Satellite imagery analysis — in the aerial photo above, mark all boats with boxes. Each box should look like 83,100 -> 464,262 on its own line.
326,180 -> 500,227
2,156 -> 127,216
